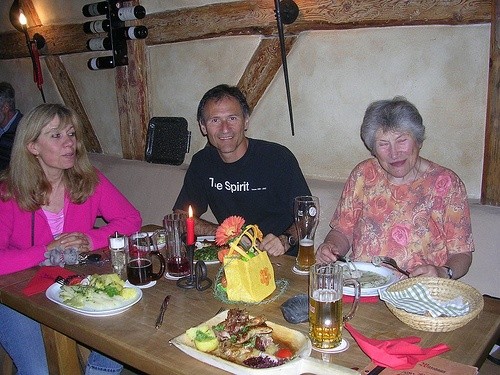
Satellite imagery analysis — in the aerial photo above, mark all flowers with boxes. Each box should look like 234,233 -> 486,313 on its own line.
215,216 -> 248,251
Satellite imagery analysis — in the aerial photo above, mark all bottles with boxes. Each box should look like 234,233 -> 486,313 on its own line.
108,231 -> 128,275
83,19 -> 110,34
86,37 -> 112,51
82,1 -> 109,17
117,5 -> 146,21
87,56 -> 114,70
123,25 -> 149,40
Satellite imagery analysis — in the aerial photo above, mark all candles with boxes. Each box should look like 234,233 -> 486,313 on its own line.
187,205 -> 195,245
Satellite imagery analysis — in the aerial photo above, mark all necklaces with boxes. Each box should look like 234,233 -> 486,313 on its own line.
387,158 -> 421,204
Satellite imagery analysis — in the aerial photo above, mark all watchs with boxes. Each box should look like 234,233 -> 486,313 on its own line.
443,265 -> 452,279
281,232 -> 296,248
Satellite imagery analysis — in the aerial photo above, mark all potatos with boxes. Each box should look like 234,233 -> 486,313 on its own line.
186,325 -> 219,352
91,273 -> 135,298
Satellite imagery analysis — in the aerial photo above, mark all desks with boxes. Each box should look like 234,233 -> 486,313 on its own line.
0,224 -> 499,374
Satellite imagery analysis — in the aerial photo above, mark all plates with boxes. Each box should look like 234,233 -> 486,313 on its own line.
45,274 -> 143,317
341,289 -> 390,296
169,309 -> 313,375
192,235 -> 220,264
126,279 -> 157,289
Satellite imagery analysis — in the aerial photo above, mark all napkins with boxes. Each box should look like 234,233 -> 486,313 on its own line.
22,266 -> 83,297
345,323 -> 451,371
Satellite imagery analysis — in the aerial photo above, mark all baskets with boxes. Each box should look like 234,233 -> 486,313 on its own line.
384,277 -> 484,332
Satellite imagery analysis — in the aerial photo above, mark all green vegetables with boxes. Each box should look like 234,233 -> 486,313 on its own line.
194,324 -> 256,348
70,280 -> 121,298
193,244 -> 225,262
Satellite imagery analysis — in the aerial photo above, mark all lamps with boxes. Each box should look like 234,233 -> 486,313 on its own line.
9,0 -> 45,104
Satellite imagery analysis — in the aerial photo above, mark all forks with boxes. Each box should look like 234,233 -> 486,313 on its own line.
378,256 -> 409,276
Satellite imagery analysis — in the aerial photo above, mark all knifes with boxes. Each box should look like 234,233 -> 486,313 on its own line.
155,295 -> 175,330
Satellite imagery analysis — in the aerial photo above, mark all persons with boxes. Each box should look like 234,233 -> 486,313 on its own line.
0,82 -> 24,172
0,103 -> 142,375
314,96 -> 475,280
173,84 -> 316,256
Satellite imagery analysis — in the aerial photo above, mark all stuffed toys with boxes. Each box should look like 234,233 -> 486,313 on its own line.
41,247 -> 79,268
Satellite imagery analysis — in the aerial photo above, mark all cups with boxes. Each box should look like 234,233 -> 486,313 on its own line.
308,261 -> 362,349
162,213 -> 192,280
127,231 -> 166,286
290,196 -> 320,275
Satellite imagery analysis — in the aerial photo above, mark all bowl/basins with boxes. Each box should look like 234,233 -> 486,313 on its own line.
337,262 -> 397,292
